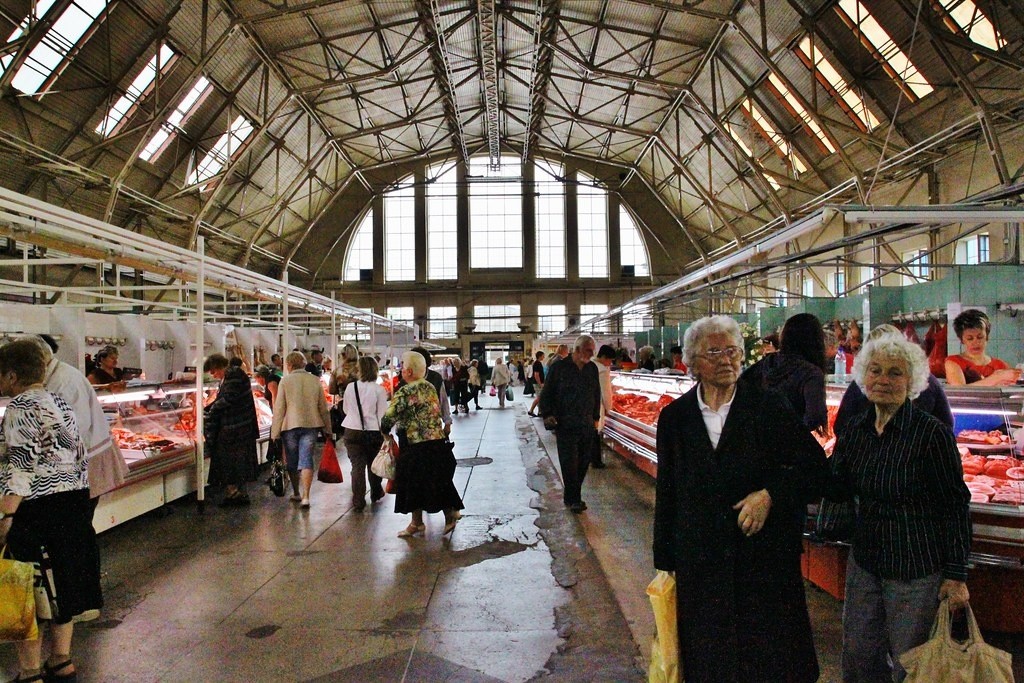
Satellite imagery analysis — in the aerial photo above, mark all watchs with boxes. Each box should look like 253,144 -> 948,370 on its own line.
0,511 -> 16,520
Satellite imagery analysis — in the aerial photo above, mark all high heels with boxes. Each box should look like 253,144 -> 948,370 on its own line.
443,508 -> 462,535
398,523 -> 426,536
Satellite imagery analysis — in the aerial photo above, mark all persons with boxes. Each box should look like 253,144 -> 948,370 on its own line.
591,345 -> 616,469
540,334 -> 601,511
13,336 -> 130,623
381,351 -> 465,535
653,314 -> 826,683
945,308 -> 1022,452
203,354 -> 260,507
398,344 -> 567,435
822,330 -> 854,374
0,339 -> 90,683
833,323 -> 955,437
809,335 -> 973,683
669,346 -> 687,373
637,345 -> 655,371
743,313 -> 827,430
253,344 -> 390,513
87,345 -> 126,419
762,335 -> 780,354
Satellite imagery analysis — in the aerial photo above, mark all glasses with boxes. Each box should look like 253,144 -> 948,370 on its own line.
692,345 -> 743,364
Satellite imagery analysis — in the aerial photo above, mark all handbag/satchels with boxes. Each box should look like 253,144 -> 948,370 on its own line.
382,435 -> 399,494
646,569 -> 685,683
371,438 -> 396,480
270,439 -> 285,497
317,437 -> 343,483
0,543 -> 38,643
898,599 -> 1015,683
523,381 -> 535,394
506,385 -> 513,401
489,386 -> 496,396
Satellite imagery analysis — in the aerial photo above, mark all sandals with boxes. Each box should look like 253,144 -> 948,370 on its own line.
7,674 -> 45,683
41,659 -> 77,683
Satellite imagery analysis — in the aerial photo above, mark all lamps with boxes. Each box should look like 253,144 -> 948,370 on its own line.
463,324 -> 477,334
554,206 -> 1024,341
517,322 -> 532,332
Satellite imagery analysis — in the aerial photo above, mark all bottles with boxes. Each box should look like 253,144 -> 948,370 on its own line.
835,348 -> 846,385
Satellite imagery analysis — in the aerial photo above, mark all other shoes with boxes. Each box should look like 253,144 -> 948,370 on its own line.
353,507 -> 363,514
72,609 -> 100,623
564,497 -> 587,515
592,462 -> 606,468
290,494 -> 301,501
370,501 -> 382,511
299,499 -> 310,509
